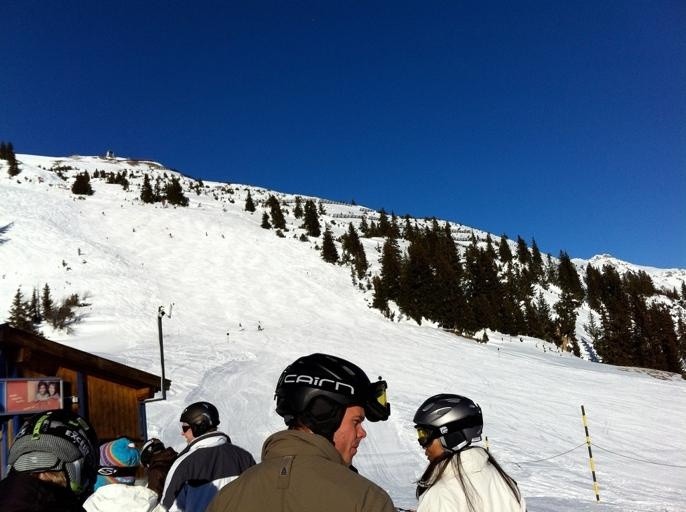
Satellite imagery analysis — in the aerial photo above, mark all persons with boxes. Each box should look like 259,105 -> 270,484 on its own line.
205,353 -> 395,512
160,401 -> 256,512
140,438 -> 179,502
82,438 -> 168,512
413,393 -> 527,512
47,382 -> 59,399
36,382 -> 50,400
0,408 -> 99,512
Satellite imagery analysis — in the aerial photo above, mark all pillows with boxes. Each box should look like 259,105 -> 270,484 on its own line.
140,437 -> 164,468
9,409 -> 100,491
180,401 -> 220,437
414,393 -> 483,452
274,353 -> 370,433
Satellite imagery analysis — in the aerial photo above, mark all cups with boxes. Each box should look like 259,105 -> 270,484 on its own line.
282,373 -> 391,422
414,414 -> 483,445
182,426 -> 191,433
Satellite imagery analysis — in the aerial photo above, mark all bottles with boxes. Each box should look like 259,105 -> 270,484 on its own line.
93,437 -> 141,491
